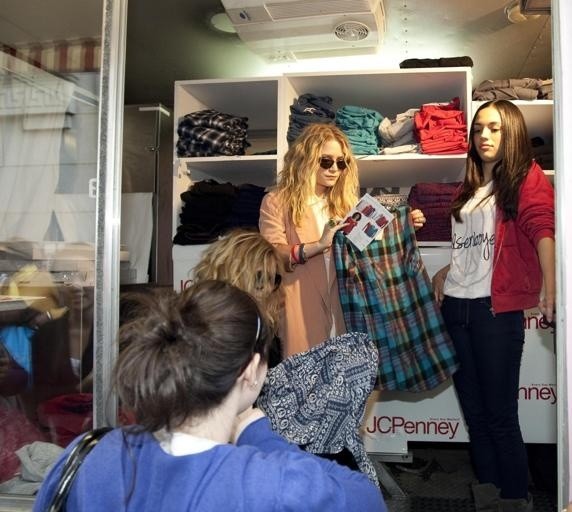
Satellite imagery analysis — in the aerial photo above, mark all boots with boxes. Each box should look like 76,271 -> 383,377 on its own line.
470,482 -> 502,512
499,493 -> 537,512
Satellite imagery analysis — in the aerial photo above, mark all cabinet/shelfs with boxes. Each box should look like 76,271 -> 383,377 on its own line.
280,67 -> 472,462
471,100 -> 559,443
173,76 -> 279,297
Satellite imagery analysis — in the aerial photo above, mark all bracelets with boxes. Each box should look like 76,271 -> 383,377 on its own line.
299,243 -> 305,264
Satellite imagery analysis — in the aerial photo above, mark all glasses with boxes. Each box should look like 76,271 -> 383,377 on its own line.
318,157 -> 348,170
254,270 -> 284,292
56,270 -> 89,282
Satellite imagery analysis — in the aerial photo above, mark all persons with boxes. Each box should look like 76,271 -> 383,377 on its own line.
187,230 -> 379,471
31,241 -> 94,433
30,278 -> 390,512
258,121 -> 426,425
1,238 -> 60,314
431,100 -> 555,512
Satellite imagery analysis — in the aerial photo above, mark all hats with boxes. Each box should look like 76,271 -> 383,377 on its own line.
0,237 -> 48,261
